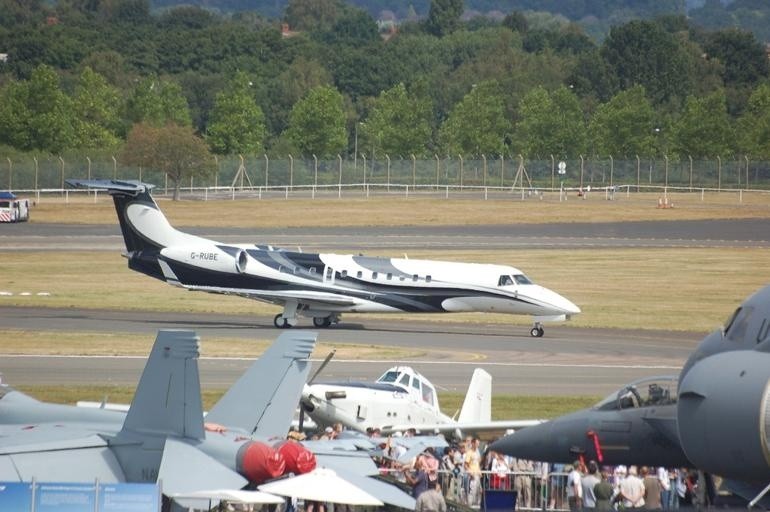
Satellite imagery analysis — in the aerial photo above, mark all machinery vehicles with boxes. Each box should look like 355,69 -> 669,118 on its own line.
1,192 -> 31,224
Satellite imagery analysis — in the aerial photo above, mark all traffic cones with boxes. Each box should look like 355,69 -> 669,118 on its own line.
657,195 -> 674,209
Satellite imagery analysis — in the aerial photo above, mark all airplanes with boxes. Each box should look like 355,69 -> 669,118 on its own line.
63,177 -> 582,337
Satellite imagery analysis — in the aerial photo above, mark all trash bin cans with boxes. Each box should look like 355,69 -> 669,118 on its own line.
480,490 -> 518,512
